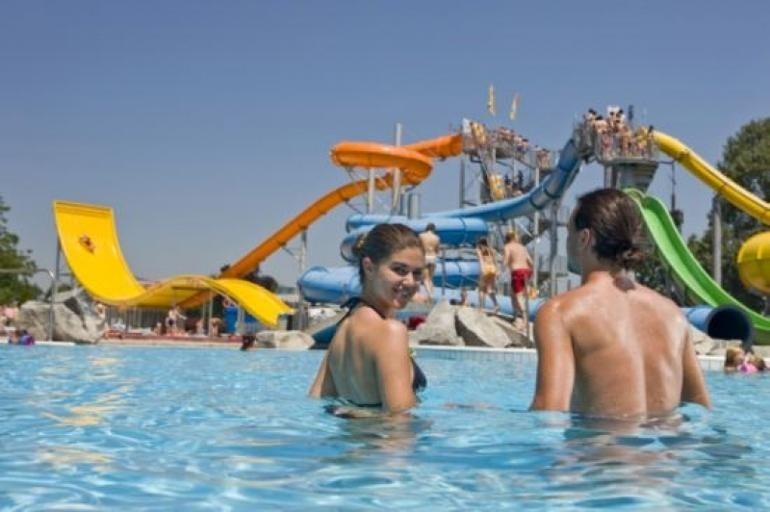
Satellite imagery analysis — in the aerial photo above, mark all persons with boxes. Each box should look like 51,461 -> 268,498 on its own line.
474,233 -> 502,315
526,185 -> 716,459
573,108 -> 657,162
496,230 -> 535,329
723,344 -> 747,372
416,223 -> 438,303
411,286 -> 425,304
725,356 -> 766,376
152,297 -> 233,338
92,301 -> 109,339
479,126 -> 553,205
458,286 -> 470,305
306,223 -> 429,439
237,333 -> 259,353
0,298 -> 33,345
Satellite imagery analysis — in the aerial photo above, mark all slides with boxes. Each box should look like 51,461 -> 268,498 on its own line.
648,125 -> 769,297
52,134 -> 463,329
626,189 -> 770,346
296,134 -> 755,347
